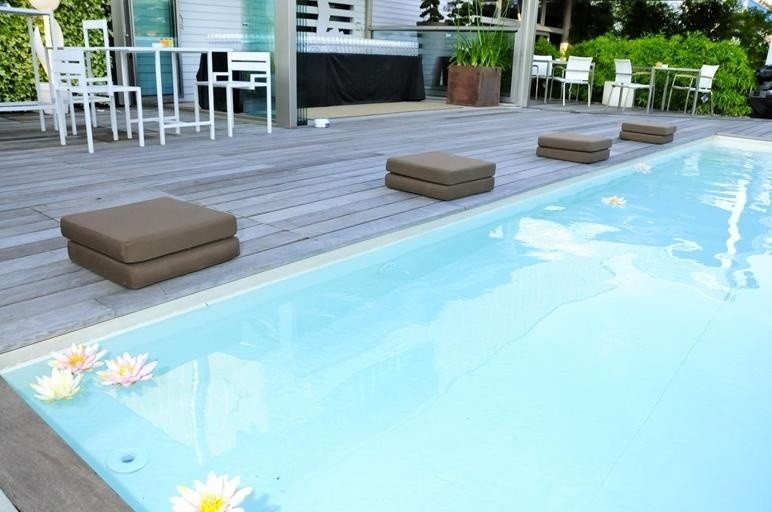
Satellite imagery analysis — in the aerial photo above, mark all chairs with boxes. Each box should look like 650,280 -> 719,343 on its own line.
531,54 -> 654,114
50,20 -> 145,154
194,52 -> 271,136
666,64 -> 719,118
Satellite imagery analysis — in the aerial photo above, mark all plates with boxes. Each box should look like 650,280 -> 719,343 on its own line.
152,43 -> 162,49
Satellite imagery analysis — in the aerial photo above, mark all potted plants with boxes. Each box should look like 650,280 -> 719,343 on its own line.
446,0 -> 509,108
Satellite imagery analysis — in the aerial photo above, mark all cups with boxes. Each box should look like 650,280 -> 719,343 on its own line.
159,37 -> 173,48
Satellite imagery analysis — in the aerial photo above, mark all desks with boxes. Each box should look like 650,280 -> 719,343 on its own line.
633,67 -> 701,115
48,46 -> 236,145
